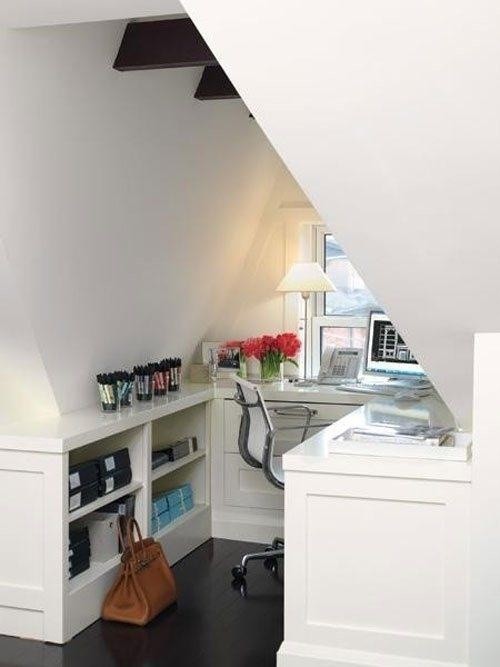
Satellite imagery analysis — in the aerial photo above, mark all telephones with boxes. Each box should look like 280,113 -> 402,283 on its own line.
318,346 -> 363,378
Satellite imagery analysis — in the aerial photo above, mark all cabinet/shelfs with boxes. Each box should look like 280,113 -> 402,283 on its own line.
0,377 -> 211,646
211,380 -> 374,545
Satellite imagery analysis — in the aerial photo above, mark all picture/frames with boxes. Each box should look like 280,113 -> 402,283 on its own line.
201,341 -> 247,378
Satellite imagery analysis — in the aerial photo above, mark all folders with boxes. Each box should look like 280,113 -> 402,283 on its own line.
69,448 -> 135,580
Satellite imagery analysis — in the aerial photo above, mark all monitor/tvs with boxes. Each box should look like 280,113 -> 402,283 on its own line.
361,308 -> 432,389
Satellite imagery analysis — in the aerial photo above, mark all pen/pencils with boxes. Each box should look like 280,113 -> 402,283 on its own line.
97,357 -> 181,410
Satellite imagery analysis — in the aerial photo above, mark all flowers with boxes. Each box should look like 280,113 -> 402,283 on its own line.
243,333 -> 302,379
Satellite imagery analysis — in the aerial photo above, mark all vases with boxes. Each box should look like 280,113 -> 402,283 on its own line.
260,351 -> 281,380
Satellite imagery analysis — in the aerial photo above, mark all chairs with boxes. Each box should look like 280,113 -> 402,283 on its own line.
229,371 -> 335,598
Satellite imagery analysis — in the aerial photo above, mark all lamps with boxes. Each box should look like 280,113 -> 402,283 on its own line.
273,261 -> 338,387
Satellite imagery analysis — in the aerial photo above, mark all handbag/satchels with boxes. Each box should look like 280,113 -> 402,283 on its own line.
101,515 -> 180,626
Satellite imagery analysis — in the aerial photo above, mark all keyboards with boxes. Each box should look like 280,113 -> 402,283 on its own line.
335,382 -> 434,397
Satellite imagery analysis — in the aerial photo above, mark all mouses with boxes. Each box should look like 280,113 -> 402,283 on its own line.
394,390 -> 420,401
